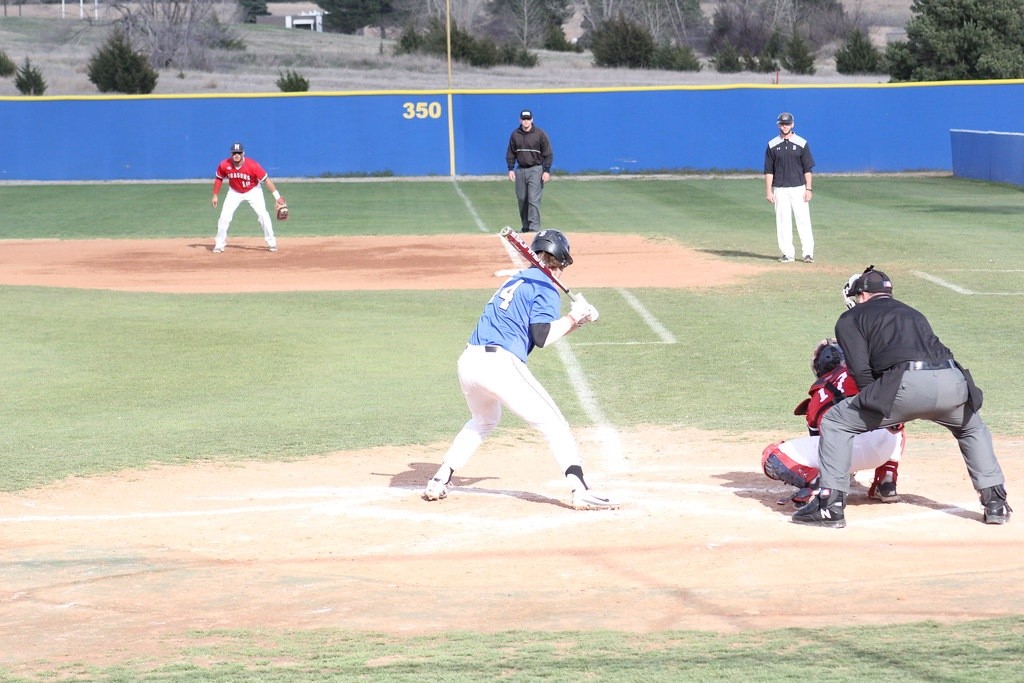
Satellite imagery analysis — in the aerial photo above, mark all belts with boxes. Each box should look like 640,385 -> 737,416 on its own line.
906,361 -> 957,370
466,345 -> 498,352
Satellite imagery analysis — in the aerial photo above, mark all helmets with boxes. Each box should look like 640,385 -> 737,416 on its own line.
531,228 -> 573,266
776,113 -> 794,125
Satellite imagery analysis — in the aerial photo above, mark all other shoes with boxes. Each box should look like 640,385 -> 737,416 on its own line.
270,247 -> 275,250
213,248 -> 224,253
804,255 -> 813,262
778,255 -> 794,262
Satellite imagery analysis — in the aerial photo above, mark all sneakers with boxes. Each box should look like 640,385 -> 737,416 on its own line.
984,501 -> 1010,524
571,488 -> 620,510
794,501 -> 847,527
871,481 -> 900,502
793,502 -> 805,510
425,478 -> 448,501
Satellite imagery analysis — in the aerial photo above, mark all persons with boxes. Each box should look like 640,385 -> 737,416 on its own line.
764,113 -> 816,264
423,231 -> 623,512
211,144 -> 289,254
506,110 -> 553,232
763,265 -> 1013,529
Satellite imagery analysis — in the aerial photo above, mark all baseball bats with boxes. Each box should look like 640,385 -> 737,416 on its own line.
500,223 -> 594,321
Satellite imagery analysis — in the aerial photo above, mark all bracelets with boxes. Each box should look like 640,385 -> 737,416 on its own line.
806,189 -> 812,191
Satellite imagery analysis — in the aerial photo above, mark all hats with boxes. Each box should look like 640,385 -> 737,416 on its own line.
848,272 -> 892,293
230,144 -> 244,152
521,109 -> 532,119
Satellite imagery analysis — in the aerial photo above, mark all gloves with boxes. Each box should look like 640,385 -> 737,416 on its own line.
570,296 -> 598,323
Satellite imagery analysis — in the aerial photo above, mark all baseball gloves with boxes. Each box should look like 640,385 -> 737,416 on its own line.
276,198 -> 288,220
